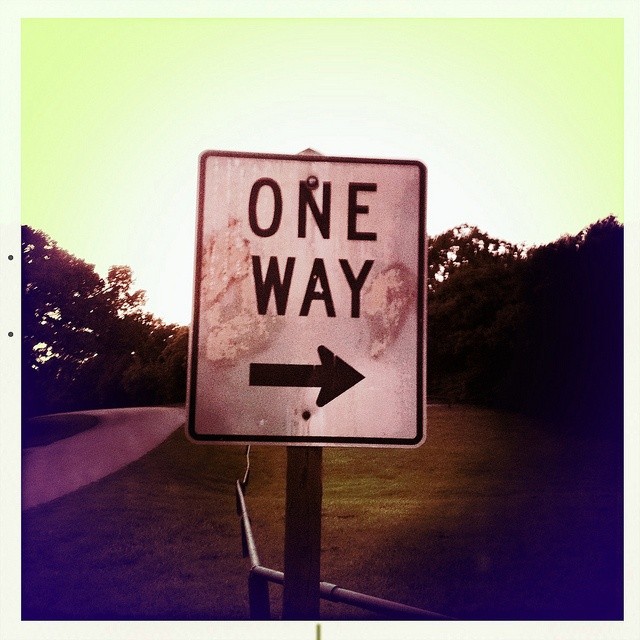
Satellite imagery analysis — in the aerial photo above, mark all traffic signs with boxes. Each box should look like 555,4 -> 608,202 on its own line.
184,150 -> 427,449
